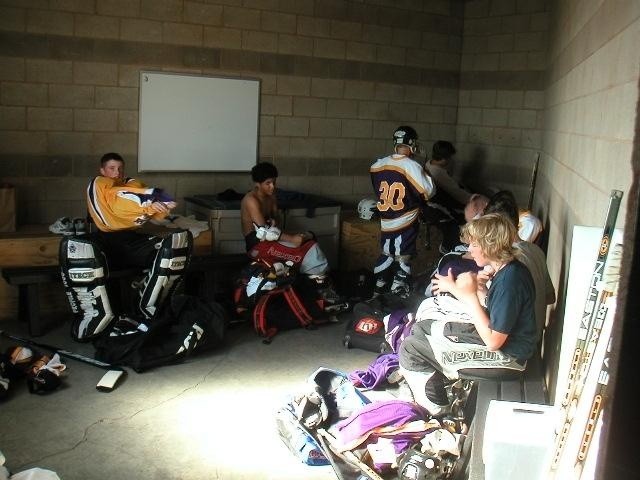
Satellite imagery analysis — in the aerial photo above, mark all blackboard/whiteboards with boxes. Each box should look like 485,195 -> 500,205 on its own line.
138,71 -> 262,174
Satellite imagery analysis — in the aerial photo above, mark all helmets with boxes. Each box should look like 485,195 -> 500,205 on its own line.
396,442 -> 460,480
393,126 -> 418,154
357,198 -> 378,220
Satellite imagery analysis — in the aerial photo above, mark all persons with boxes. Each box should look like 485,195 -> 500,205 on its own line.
423,139 -> 473,255
396,212 -> 538,417
57,151 -> 194,342
238,160 -> 350,310
424,194 -> 490,297
492,190 -> 549,260
413,197 -> 557,361
368,125 -> 440,298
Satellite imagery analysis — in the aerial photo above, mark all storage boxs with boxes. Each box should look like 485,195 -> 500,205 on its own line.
0,185 -> 20,233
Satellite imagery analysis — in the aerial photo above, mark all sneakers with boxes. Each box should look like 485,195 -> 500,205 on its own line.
319,288 -> 342,304
48,216 -> 86,236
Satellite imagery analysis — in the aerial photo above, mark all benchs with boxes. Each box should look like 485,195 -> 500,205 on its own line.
449,347 -> 548,479
2,252 -> 251,338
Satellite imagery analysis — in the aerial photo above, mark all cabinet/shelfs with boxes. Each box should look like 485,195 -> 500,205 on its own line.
183,191 -> 342,298
0,217 -> 213,318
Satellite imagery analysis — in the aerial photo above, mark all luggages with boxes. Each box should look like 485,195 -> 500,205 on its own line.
343,292 -> 409,354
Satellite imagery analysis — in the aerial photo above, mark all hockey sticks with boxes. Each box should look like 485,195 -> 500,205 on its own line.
0,331 -> 124,391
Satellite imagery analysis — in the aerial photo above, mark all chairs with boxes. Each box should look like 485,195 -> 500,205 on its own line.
413,200 -> 464,288
